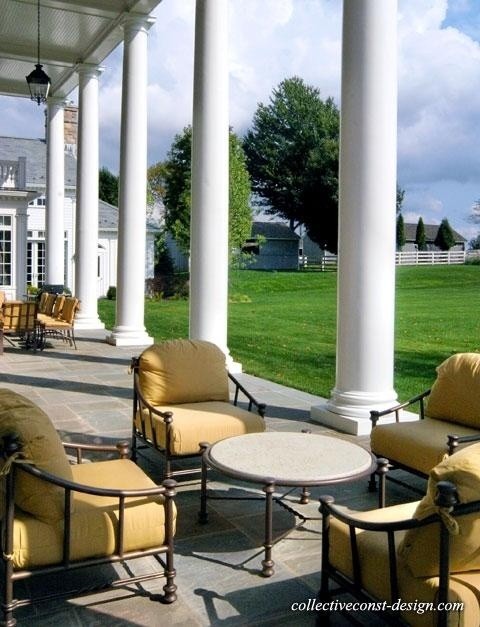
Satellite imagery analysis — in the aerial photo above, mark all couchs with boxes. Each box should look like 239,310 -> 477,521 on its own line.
369,352 -> 480,493
319,441 -> 480,626
131,337 -> 266,479
0,387 -> 179,626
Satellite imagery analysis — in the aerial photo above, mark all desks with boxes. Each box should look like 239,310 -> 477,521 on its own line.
198,428 -> 390,576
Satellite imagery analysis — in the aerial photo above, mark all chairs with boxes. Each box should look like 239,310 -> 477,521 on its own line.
0,291 -> 81,354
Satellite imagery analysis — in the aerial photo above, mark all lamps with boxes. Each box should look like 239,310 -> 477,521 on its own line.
26,2 -> 53,104
